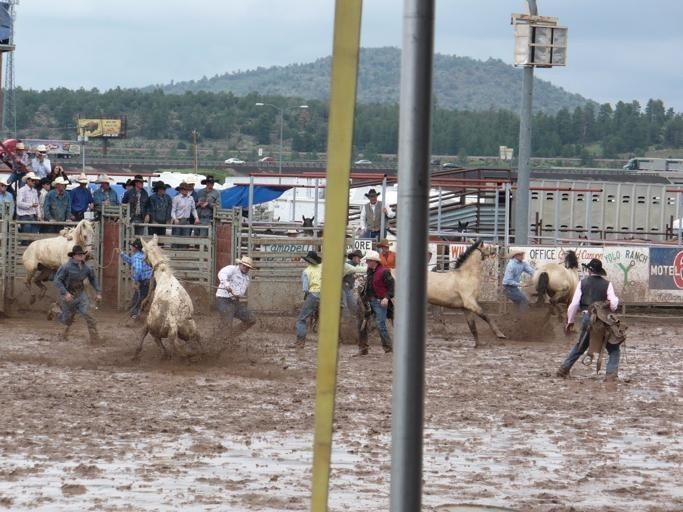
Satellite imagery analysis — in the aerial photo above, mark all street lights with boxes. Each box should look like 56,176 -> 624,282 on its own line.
254,102 -> 309,174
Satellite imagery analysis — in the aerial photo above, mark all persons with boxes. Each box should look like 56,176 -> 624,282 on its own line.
501,248 -> 536,314
359,189 -> 394,238
215,256 -> 257,340
53,245 -> 102,344
295,239 -> 396,357
557,259 -> 620,380
0,143 -> 222,248
114,239 -> 153,320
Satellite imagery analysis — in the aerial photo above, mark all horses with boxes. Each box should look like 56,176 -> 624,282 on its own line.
132,234 -> 209,365
440,221 -> 469,242
21,219 -> 96,305
385,237 -> 507,348
533,250 -> 579,336
286,215 -> 315,236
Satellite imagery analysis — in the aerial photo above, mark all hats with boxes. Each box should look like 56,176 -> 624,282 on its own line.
15,143 -> 24,150
511,249 -> 525,258
37,145 -> 46,152
363,250 -> 381,261
67,245 -> 88,256
348,250 -> 364,260
129,239 -> 143,252
376,239 -> 393,248
301,251 -> 322,265
234,256 -> 256,271
582,259 -> 606,277
364,189 -> 379,197
0,171 -> 219,192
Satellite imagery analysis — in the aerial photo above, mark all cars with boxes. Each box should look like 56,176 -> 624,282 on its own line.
257,156 -> 276,164
442,162 -> 464,168
354,160 -> 373,165
225,157 -> 246,165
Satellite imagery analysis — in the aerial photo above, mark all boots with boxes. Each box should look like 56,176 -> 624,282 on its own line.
286,336 -> 305,349
380,335 -> 392,353
352,335 -> 368,358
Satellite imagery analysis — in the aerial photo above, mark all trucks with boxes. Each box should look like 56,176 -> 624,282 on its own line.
621,158 -> 683,171
